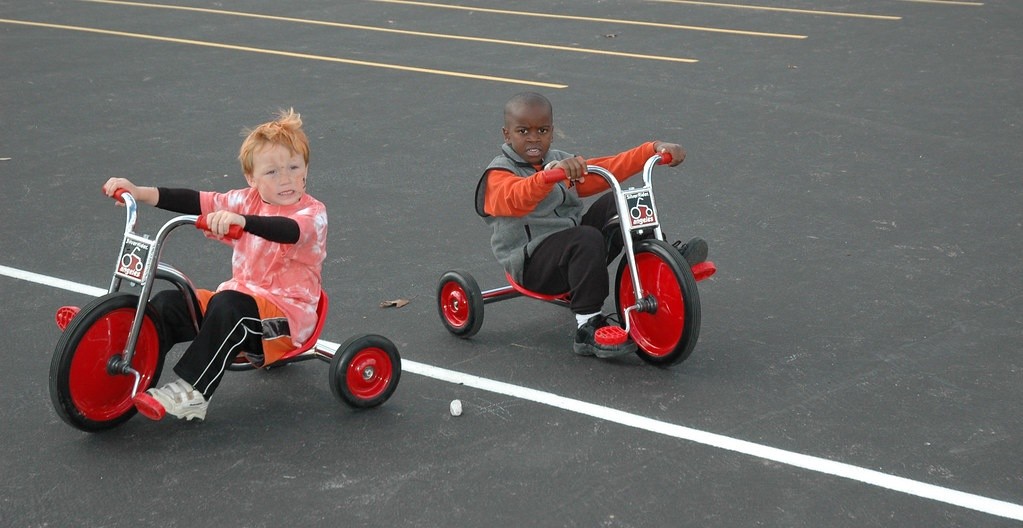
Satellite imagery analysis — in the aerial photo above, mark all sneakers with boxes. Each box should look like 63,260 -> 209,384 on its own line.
672,236 -> 708,270
573,312 -> 637,358
146,378 -> 214,424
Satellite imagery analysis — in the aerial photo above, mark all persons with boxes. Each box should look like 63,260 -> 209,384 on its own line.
474,92 -> 709,360
103,108 -> 327,422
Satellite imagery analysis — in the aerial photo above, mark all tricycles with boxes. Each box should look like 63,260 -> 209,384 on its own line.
47,187 -> 401,435
435,147 -> 715,370
